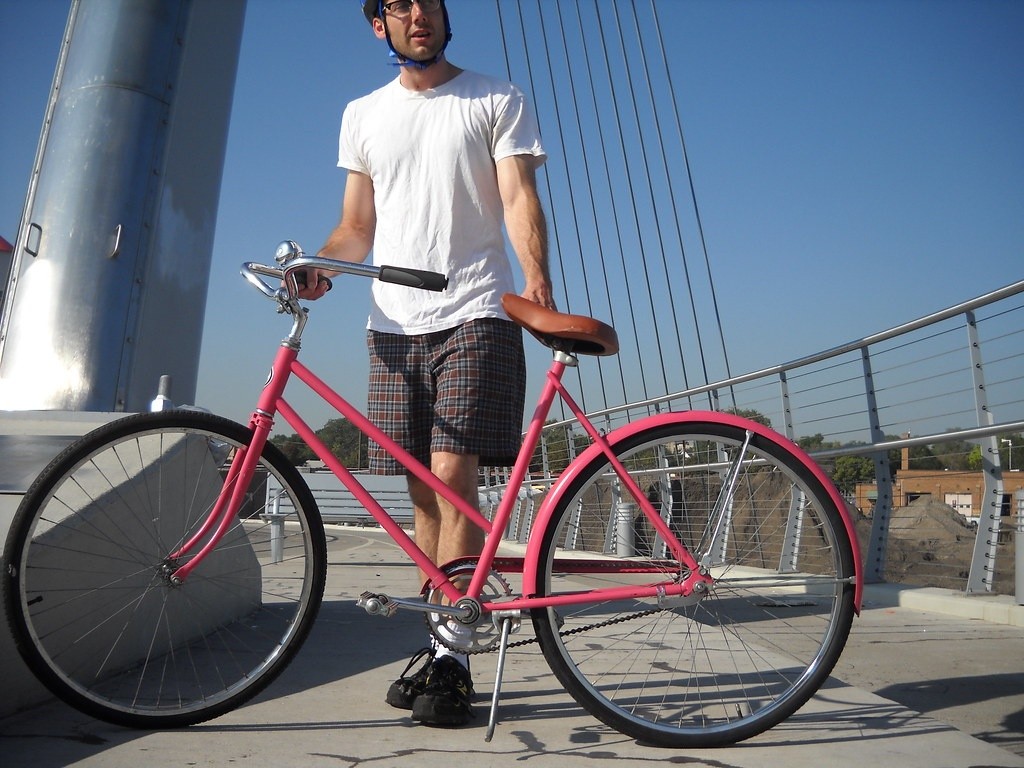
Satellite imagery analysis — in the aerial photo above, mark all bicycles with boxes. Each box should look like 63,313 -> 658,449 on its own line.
1,240 -> 864,746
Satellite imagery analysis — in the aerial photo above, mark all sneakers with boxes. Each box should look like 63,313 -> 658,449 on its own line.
386,646 -> 438,710
411,654 -> 476,727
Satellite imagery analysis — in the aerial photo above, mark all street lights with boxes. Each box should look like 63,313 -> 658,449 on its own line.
1000,438 -> 1012,472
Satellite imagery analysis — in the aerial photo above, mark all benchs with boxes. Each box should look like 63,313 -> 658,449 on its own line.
259,472 -> 416,563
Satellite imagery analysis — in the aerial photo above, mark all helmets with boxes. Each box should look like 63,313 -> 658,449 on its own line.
359,0 -> 379,27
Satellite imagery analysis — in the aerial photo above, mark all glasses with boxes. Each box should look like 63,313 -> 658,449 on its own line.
382,0 -> 440,12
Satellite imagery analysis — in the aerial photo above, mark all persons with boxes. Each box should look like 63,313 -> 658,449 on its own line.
281,0 -> 559,727
